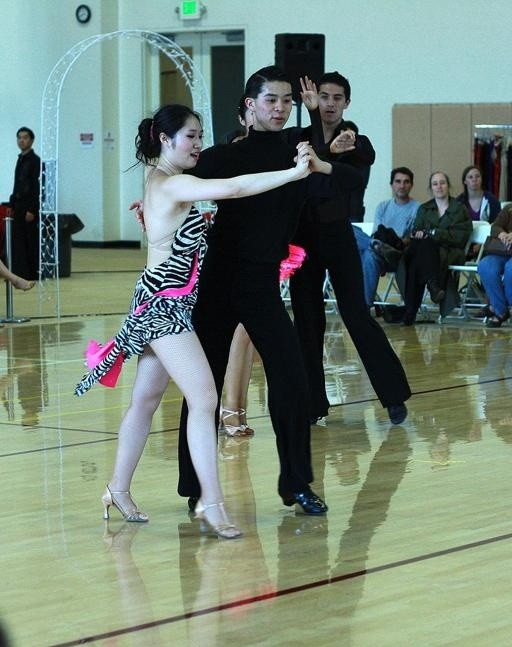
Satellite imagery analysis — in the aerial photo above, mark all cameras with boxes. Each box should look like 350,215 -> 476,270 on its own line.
413,227 -> 440,237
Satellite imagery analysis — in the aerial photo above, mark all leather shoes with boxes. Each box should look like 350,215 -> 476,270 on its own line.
218,405 -> 254,438
399,283 -> 509,328
387,400 -> 407,425
282,482 -> 328,516
186,496 -> 197,510
310,407 -> 329,425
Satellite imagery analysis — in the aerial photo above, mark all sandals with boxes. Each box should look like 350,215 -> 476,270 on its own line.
12,275 -> 35,291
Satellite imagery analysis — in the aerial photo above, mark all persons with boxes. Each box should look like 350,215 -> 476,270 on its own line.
130,65 -> 359,513
0,259 -> 36,290
7,126 -> 45,280
456,166 -> 501,223
13,325 -> 50,429
478,203 -> 512,327
351,166 -> 421,318
218,75 -> 355,437
285,70 -> 412,425
402,171 -> 474,326
73,104 -> 314,540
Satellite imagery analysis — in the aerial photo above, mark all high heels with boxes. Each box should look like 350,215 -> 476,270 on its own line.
194,499 -> 246,539
102,482 -> 151,522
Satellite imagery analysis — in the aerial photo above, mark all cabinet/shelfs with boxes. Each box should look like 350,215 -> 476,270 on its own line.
392,104 -> 472,199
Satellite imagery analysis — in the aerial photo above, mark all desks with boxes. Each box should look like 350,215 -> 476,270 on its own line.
0,213 -> 85,279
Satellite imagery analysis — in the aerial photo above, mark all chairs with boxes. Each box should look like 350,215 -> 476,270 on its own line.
278,202 -> 511,325
